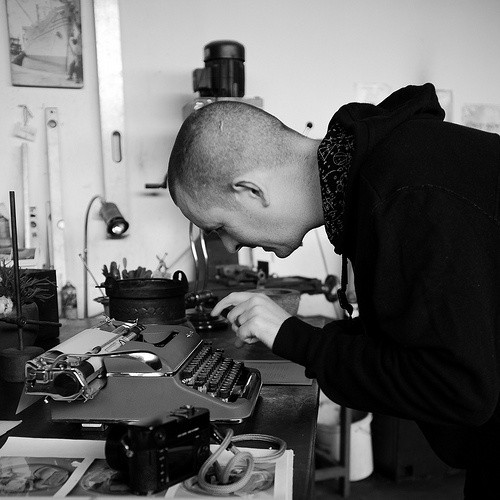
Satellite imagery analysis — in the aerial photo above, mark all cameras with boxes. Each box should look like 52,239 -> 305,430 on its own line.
105,404 -> 212,496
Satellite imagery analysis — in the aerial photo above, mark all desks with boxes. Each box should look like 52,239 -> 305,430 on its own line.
0,294 -> 328,499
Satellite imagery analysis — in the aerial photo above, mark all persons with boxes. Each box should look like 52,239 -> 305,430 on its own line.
167,84 -> 500,500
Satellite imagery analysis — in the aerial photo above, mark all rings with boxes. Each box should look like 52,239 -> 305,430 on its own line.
234,315 -> 242,329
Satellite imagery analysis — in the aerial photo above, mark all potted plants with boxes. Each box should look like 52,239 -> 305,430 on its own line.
0,257 -> 58,346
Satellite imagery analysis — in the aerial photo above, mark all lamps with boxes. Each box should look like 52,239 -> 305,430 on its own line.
84,196 -> 130,318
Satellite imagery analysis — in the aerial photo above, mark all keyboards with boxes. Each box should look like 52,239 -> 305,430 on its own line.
181,340 -> 244,401
